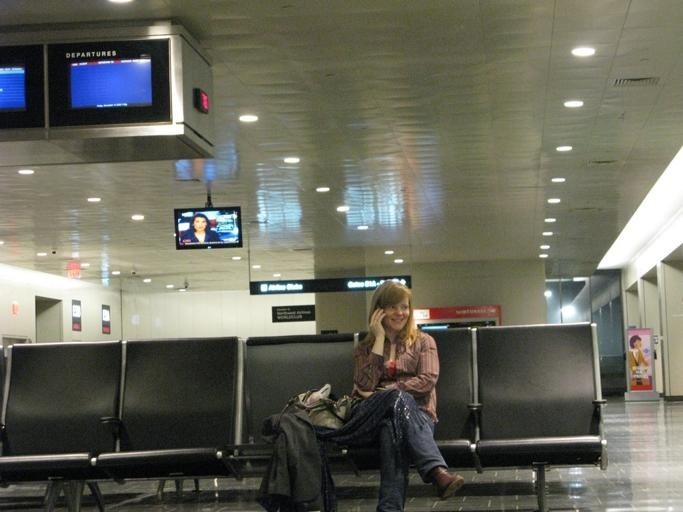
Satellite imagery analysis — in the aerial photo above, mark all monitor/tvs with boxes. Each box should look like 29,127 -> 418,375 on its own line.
0,44 -> 44,129
173,205 -> 243,250
46,38 -> 169,127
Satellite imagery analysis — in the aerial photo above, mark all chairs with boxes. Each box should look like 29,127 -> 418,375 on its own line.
474,323 -> 606,510
0,339 -> 123,512
227,333 -> 356,464
99,336 -> 239,500
356,326 -> 474,468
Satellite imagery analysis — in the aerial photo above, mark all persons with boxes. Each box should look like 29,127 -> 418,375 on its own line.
350,280 -> 464,512
629,334 -> 650,385
182,213 -> 223,243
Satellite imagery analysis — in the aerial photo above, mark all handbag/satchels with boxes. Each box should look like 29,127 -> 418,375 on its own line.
272,385 -> 357,437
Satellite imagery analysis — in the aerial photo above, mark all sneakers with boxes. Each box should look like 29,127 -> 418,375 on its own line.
429,466 -> 465,499
